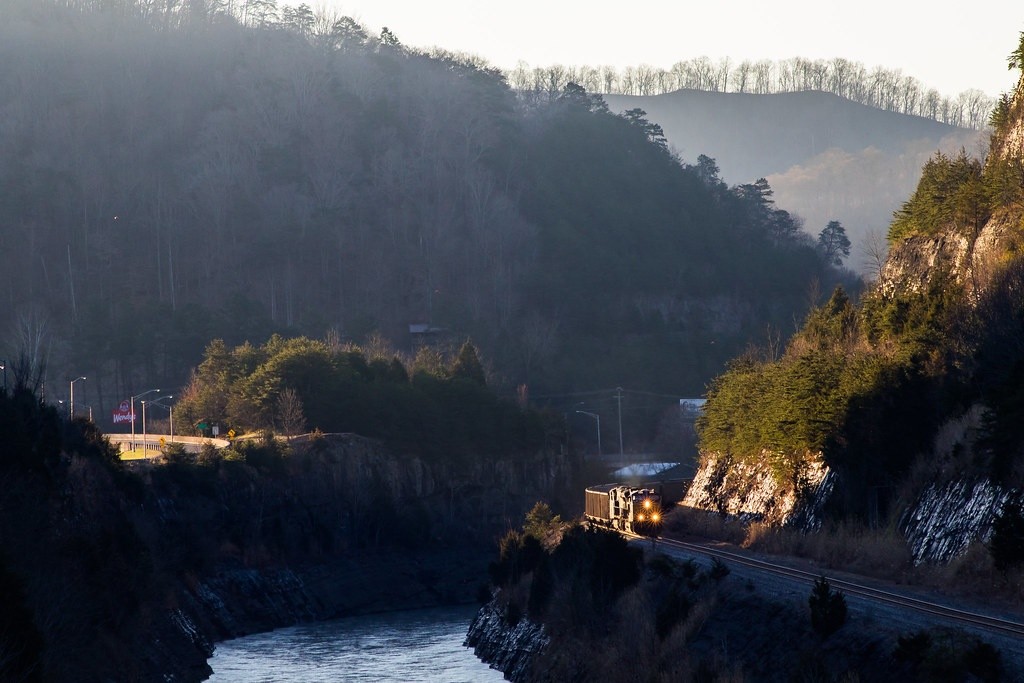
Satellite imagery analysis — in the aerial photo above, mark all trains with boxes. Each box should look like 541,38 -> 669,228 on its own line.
585,478 -> 697,536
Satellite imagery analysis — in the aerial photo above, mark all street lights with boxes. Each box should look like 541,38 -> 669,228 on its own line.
575,410 -> 601,461
58,399 -> 93,425
69,375 -> 87,423
142,394 -> 175,459
131,388 -> 161,452
